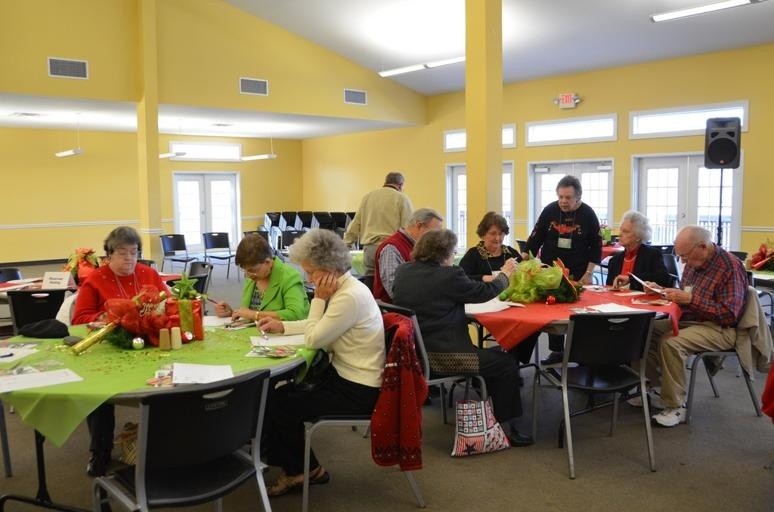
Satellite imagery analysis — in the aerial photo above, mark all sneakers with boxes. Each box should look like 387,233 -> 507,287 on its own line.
87,456 -> 105,475
627,392 -> 659,407
652,407 -> 691,426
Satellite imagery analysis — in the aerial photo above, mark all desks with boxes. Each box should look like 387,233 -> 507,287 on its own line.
276,246 -> 366,297
596,244 -> 624,284
1,322 -> 324,510
742,261 -> 774,337
1,274 -> 183,334
470,285 -> 683,433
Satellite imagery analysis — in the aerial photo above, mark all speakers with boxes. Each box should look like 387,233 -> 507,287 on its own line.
705,118 -> 741,169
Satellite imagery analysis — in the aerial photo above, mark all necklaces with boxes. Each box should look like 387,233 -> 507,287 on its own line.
623,254 -> 638,264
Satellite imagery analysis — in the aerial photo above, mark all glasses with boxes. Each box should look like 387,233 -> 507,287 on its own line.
671,242 -> 703,259
240,264 -> 265,277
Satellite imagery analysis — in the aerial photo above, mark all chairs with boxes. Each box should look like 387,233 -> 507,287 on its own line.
684,288 -> 764,427
7,290 -> 76,332
303,305 -> 427,508
381,301 -> 488,425
189,262 -> 213,316
158,233 -> 199,274
202,233 -> 241,280
530,312 -> 659,479
1,268 -> 23,280
89,368 -> 277,512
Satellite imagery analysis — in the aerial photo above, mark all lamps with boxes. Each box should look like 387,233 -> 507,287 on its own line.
380,65 -> 423,78
159,152 -> 185,160
55,151 -> 82,157
240,155 -> 278,161
425,57 -> 465,68
649,0 -> 760,24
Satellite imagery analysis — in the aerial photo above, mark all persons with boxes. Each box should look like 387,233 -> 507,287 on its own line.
460,211 -> 525,302
372,208 -> 446,340
72,227 -> 183,477
214,226 -> 309,445
604,213 -> 668,398
253,231 -> 387,491
341,172 -> 413,280
626,226 -> 747,429
391,228 -> 537,450
512,178 -> 602,364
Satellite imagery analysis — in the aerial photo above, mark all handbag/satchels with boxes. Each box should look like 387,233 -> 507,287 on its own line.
451,396 -> 512,457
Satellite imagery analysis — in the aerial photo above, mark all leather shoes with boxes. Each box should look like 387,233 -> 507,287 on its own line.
493,413 -> 534,446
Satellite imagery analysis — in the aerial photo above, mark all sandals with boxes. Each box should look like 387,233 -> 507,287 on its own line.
306,466 -> 329,484
261,472 -> 306,497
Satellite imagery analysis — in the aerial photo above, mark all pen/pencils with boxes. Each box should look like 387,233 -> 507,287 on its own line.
254,320 -> 268,340
207,297 -> 231,313
500,257 -> 519,271
0,353 -> 13,358
